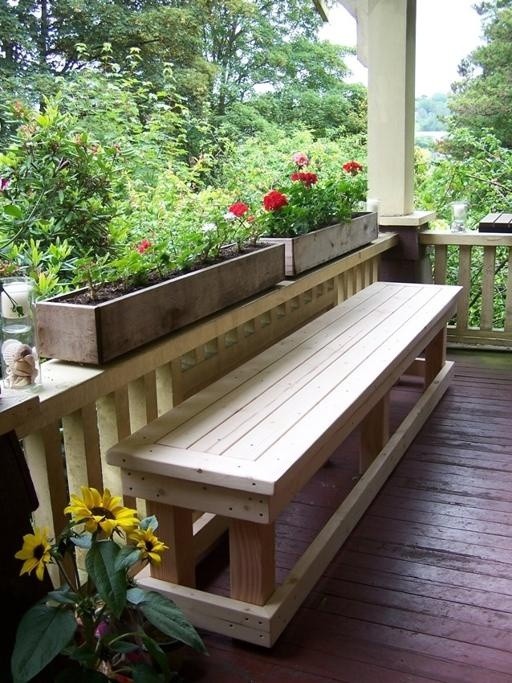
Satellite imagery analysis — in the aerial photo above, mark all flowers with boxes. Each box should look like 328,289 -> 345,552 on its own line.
10,486 -> 210,682
256,150 -> 370,238
72,183 -> 256,299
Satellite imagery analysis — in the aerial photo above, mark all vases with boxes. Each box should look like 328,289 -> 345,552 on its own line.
251,210 -> 379,277
142,620 -> 184,671
33,241 -> 286,367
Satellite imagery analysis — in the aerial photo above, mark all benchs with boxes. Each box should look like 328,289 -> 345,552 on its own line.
105,281 -> 465,649
